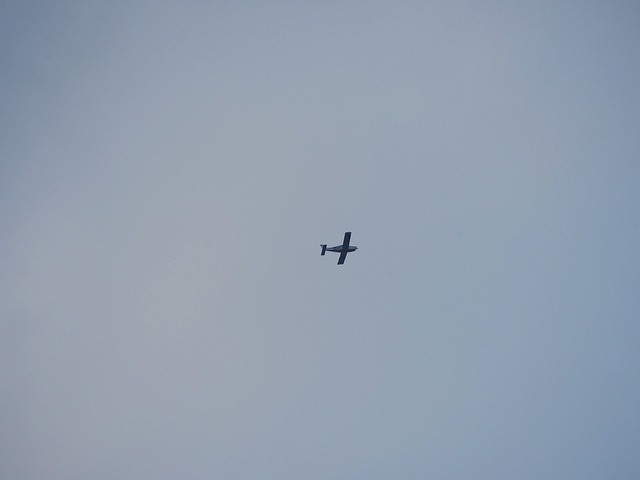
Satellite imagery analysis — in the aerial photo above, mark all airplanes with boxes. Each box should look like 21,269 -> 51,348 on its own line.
320,232 -> 358,265
